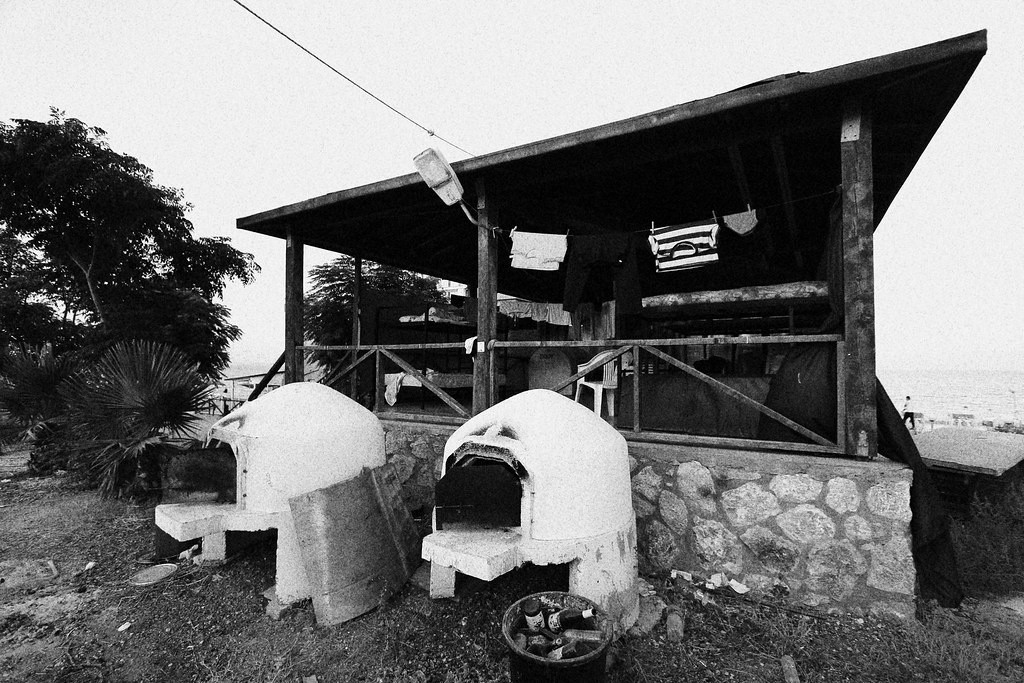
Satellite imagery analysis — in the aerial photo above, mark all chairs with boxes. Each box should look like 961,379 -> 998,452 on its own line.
574,349 -> 633,418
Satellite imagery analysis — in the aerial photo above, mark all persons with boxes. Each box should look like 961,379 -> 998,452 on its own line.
901,396 -> 916,429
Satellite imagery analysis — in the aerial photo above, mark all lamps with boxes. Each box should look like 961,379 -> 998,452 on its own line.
412,148 -> 502,234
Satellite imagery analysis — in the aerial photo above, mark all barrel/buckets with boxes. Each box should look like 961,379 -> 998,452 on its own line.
503,591 -> 613,683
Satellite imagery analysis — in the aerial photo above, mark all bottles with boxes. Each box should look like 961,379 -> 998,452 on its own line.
515,598 -> 606,660
